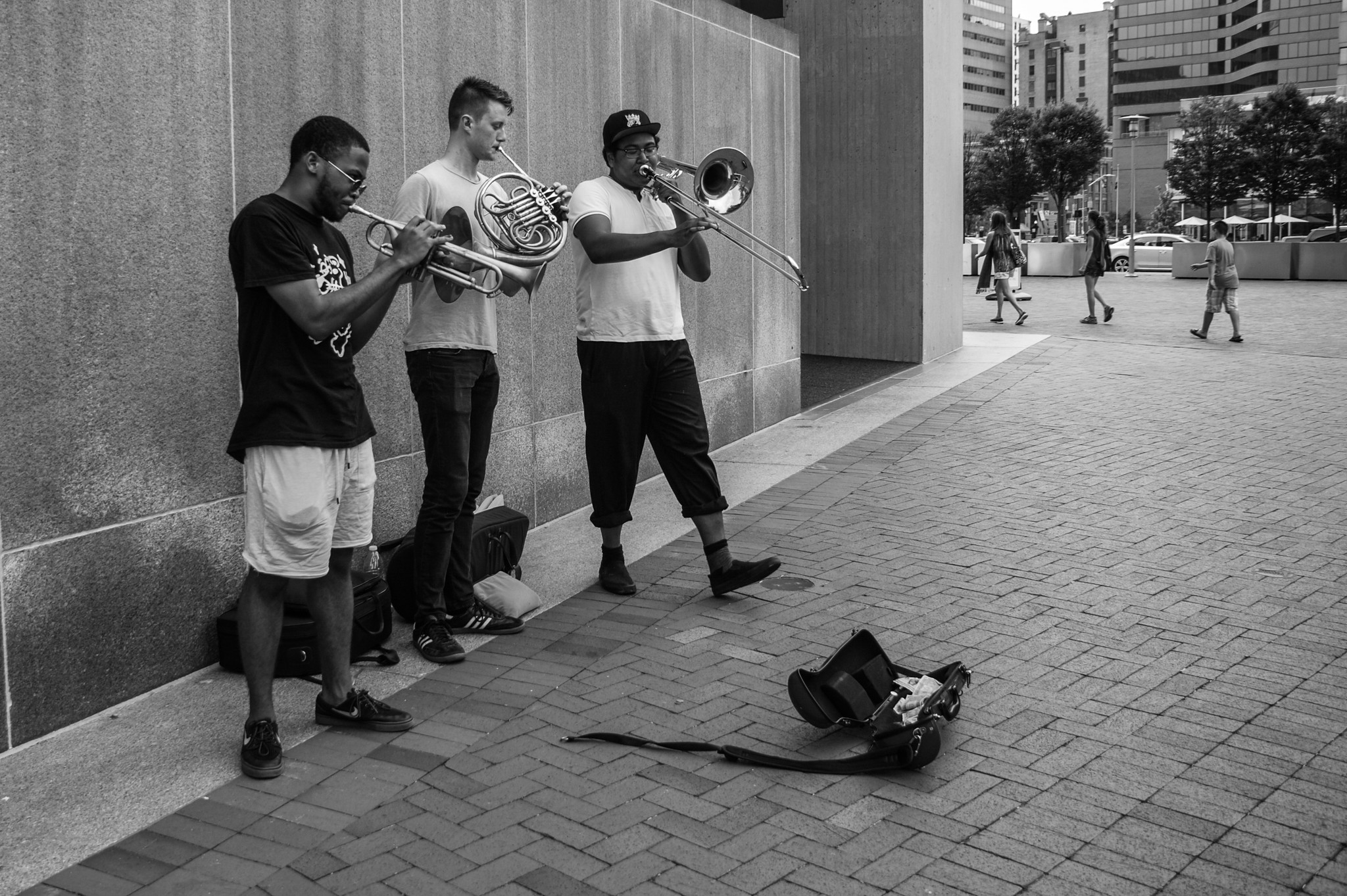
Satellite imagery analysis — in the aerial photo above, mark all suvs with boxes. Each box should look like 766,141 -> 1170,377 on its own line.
1303,226 -> 1347,243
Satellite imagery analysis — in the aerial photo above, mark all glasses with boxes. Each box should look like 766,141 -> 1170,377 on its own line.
318,152 -> 367,195
617,145 -> 659,158
989,218 -> 992,221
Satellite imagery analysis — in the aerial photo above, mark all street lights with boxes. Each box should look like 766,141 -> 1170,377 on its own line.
1118,115 -> 1151,277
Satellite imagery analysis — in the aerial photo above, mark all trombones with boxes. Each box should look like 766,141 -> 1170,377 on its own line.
637,146 -> 810,292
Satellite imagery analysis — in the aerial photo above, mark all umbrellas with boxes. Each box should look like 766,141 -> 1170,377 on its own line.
1252,213 -> 1309,241
1174,216 -> 1214,240
1294,216 -> 1330,223
1211,215 -> 1256,242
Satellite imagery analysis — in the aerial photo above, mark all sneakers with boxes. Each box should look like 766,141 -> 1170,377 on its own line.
445,601 -> 525,634
241,717 -> 284,779
413,614 -> 465,662
315,687 -> 413,732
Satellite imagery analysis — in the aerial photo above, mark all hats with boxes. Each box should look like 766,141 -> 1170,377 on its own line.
603,110 -> 661,145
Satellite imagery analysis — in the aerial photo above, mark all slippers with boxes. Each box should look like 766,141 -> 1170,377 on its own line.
1229,337 -> 1244,342
1190,328 -> 1207,339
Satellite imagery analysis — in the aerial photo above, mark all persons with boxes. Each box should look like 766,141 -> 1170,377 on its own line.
1258,234 -> 1266,241
384,77 -> 571,666
974,211 -> 1028,325
225,113 -> 454,784
1078,210 -> 1114,324
1189,220 -> 1244,343
569,109 -> 782,596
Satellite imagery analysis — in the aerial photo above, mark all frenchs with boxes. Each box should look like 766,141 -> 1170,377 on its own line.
428,149 -> 569,303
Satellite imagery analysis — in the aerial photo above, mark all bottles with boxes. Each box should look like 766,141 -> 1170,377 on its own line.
363,545 -> 393,613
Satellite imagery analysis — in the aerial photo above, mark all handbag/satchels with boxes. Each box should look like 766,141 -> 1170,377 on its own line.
216,570 -> 400,685
1011,247 -> 1027,267
1102,241 -> 1112,271
377,505 -> 529,620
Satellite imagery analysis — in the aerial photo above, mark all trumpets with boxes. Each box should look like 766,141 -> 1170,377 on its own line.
345,202 -> 548,298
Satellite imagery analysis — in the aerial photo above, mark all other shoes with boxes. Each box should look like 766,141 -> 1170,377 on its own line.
599,560 -> 637,594
708,556 -> 781,598
1104,306 -> 1114,322
1015,312 -> 1028,325
990,318 -> 1004,323
1080,316 -> 1097,324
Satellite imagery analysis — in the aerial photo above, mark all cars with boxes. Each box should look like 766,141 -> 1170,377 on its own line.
1281,235 -> 1307,242
1021,235 -> 1131,245
1108,233 -> 1200,272
